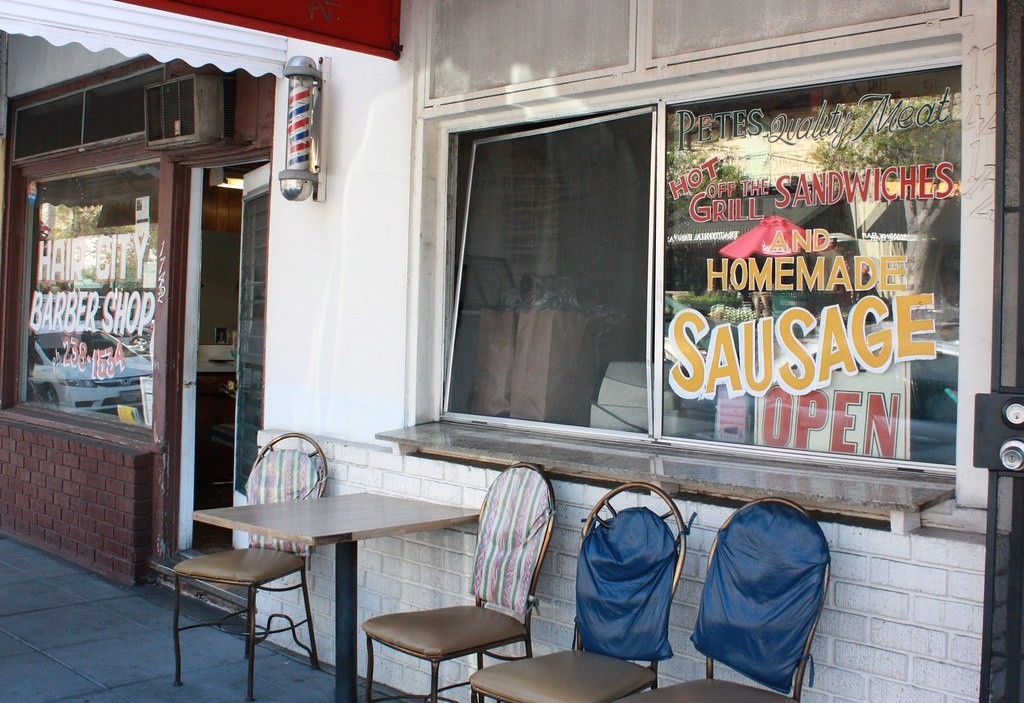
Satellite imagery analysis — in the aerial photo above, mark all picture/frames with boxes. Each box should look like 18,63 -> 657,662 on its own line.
461,255 -> 515,309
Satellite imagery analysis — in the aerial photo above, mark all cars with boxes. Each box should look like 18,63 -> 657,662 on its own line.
27,330 -> 154,410
37,295 -> 154,353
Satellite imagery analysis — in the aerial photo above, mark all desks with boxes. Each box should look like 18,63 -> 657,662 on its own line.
191,491 -> 482,703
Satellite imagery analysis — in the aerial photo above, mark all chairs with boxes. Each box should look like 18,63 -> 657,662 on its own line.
617,497 -> 832,702
470,480 -> 696,703
360,461 -> 556,703
171,432 -> 327,703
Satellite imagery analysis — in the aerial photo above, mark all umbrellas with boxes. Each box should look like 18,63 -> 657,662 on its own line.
719,214 -> 832,261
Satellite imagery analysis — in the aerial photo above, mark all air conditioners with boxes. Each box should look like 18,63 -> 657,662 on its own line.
141,73 -> 237,153
